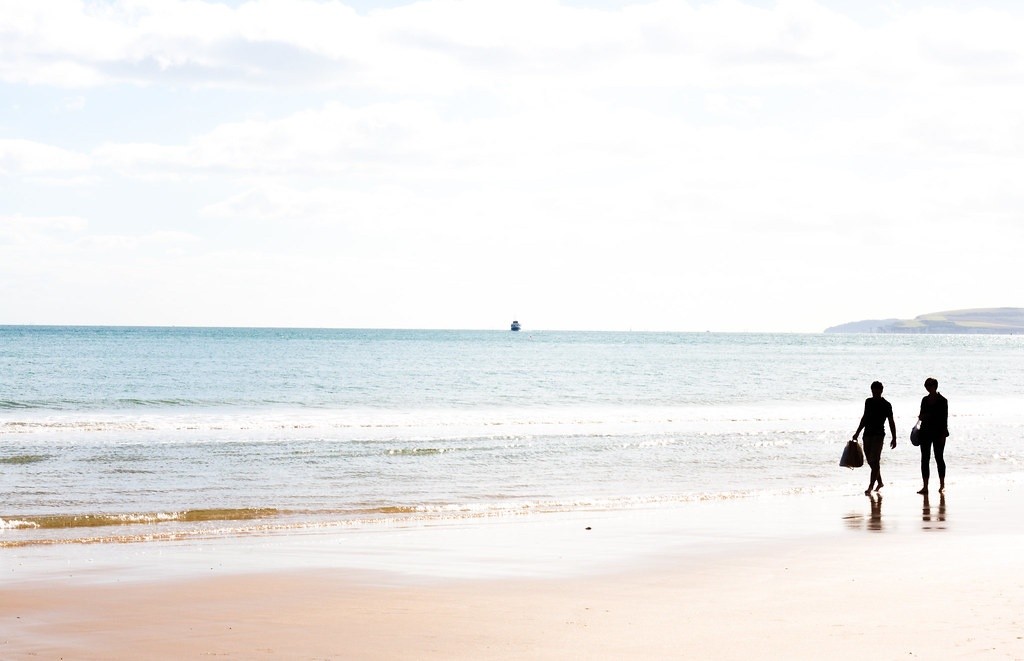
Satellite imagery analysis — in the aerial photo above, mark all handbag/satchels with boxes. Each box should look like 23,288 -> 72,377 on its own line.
910,420 -> 922,446
840,438 -> 864,467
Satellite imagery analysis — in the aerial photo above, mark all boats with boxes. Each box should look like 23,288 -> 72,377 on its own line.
511,321 -> 521,331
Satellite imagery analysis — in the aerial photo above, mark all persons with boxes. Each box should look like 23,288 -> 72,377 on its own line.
917,378 -> 949,494
853,380 -> 896,493
